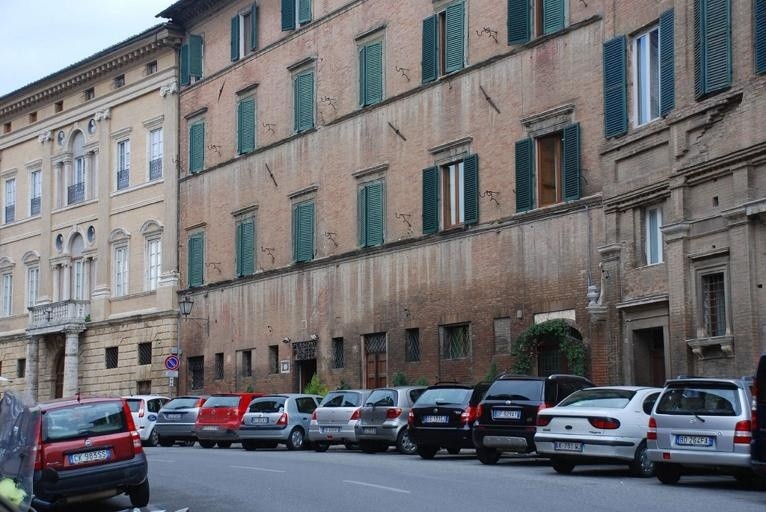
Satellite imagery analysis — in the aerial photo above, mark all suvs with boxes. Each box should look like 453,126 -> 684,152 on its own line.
10,395 -> 152,510
407,381 -> 493,460
646,354 -> 765,486
471,373 -> 600,466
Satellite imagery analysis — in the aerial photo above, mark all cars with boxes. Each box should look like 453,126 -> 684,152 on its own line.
153,394 -> 209,448
123,396 -> 172,447
532,384 -> 688,478
353,384 -> 428,455
307,389 -> 374,453
194,392 -> 266,449
237,392 -> 326,451
